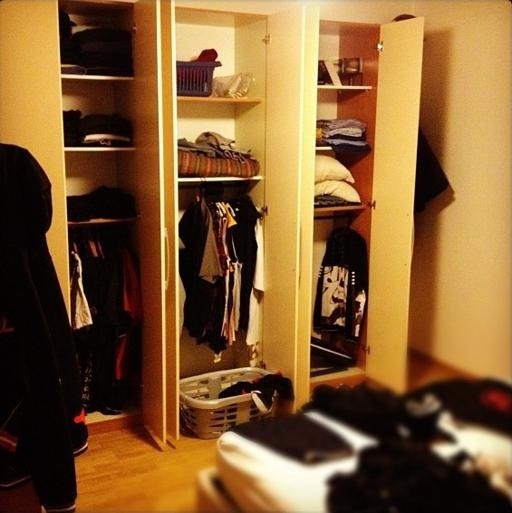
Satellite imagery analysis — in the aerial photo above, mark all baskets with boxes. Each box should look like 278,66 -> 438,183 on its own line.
175,366 -> 277,440
175,60 -> 221,96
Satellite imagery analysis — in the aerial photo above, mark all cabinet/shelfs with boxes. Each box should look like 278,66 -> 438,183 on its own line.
0,0 -> 426,445
